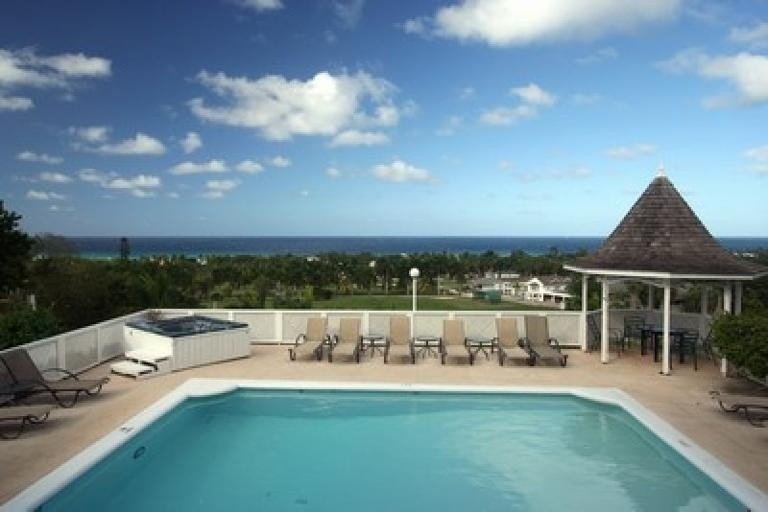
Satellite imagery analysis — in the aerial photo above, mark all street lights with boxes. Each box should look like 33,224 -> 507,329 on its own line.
408,267 -> 420,338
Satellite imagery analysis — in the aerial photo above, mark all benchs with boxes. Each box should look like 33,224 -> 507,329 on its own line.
0,404 -> 59,441
707,388 -> 767,426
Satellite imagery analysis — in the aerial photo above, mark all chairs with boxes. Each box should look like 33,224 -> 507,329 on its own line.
289,308 -> 571,370
0,346 -> 112,408
584,310 -> 704,371
